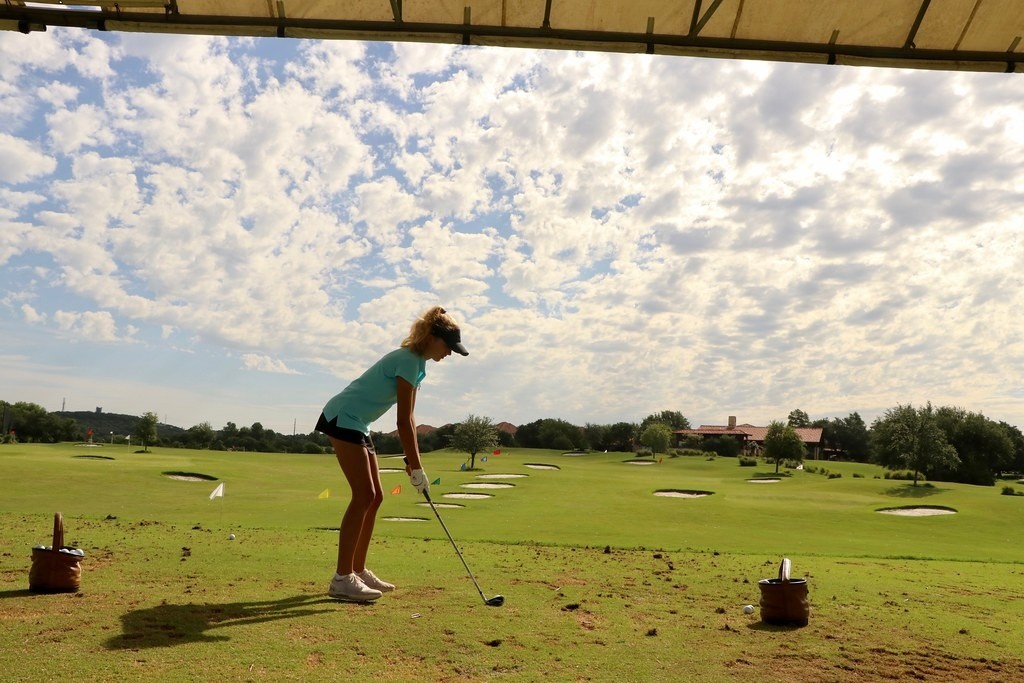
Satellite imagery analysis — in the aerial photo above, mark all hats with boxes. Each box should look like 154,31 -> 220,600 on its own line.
431,325 -> 469,356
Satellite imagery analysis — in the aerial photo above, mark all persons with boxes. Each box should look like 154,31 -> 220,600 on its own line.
314,306 -> 469,601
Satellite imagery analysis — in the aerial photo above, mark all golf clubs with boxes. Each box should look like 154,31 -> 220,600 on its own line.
403,457 -> 504,607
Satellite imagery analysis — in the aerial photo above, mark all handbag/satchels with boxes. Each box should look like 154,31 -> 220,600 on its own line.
758,558 -> 809,625
28,513 -> 84,592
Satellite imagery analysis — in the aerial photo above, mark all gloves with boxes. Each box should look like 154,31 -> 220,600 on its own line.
410,468 -> 429,495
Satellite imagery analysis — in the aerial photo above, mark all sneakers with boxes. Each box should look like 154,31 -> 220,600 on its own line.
328,573 -> 382,600
353,569 -> 394,592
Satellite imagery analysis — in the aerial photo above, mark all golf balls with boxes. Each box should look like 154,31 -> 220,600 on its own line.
229,534 -> 236,540
36,546 -> 84,556
744,605 -> 754,614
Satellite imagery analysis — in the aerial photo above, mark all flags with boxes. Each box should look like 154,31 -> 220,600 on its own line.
392,485 -> 401,494
494,450 -> 500,455
481,456 -> 486,461
318,489 -> 328,499
209,483 -> 223,499
432,478 -> 440,484
